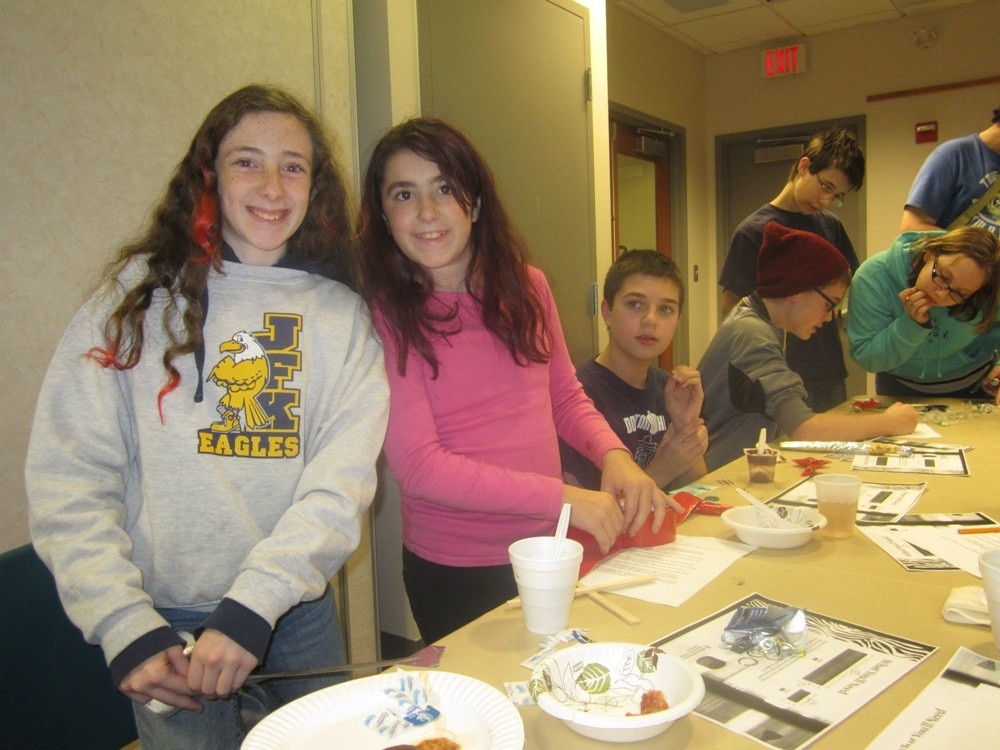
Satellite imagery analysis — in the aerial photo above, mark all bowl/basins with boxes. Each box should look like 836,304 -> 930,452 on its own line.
724,506 -> 826,550
530,642 -> 705,743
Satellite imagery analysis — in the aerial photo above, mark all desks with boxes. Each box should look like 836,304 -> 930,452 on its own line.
374,388 -> 999,750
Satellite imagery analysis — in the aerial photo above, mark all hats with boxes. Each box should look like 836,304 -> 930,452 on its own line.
757,221 -> 849,298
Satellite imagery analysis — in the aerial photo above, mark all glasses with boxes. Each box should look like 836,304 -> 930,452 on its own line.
813,286 -> 840,312
932,252 -> 973,305
809,154 -> 847,204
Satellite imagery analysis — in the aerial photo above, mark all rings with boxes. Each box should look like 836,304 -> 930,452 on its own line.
912,303 -> 919,310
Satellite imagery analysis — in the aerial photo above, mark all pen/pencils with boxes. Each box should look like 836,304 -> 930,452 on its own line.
958,528 -> 1000,534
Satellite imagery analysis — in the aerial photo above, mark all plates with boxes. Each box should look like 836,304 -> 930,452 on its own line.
236,669 -> 525,750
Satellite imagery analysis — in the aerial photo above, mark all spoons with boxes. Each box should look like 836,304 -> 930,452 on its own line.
736,486 -> 801,529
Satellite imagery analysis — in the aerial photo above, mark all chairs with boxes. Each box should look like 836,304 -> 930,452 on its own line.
0,541 -> 139,750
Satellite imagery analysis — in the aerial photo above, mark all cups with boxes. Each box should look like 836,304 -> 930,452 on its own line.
745,449 -> 777,484
813,471 -> 861,539
509,535 -> 585,636
979,550 -> 1000,654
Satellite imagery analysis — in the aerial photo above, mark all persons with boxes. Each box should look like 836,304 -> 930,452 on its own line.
554,248 -> 713,512
900,107 -> 1000,236
718,128 -> 866,415
848,223 -> 1000,404
353,117 -> 669,651
687,220 -> 923,473
26,82 -> 392,749
